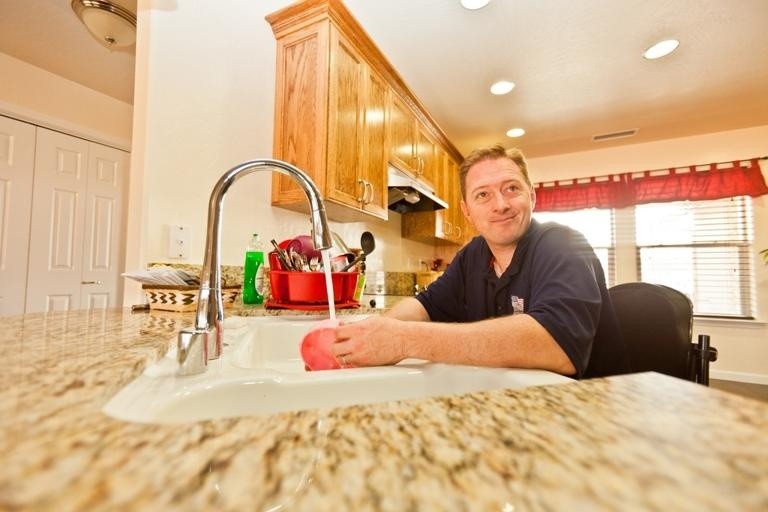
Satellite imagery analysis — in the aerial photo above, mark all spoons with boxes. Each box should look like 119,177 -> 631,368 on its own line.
339,231 -> 376,272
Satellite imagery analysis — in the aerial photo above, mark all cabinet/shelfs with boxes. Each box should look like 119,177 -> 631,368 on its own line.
401,127 -> 469,245
264,1 -> 391,223
388,63 -> 438,195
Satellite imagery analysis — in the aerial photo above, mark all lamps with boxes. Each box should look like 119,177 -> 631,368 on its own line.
71,0 -> 136,49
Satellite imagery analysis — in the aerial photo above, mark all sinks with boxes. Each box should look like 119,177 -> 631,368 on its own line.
100,315 -> 579,425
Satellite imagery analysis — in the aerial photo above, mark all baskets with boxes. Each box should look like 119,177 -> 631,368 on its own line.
142,284 -> 241,312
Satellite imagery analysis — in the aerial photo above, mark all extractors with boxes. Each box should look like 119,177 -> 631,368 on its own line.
388,163 -> 449,215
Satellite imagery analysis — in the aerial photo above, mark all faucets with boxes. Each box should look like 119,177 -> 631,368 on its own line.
195,158 -> 335,360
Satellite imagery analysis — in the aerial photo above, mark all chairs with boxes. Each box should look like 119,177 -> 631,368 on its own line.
608,282 -> 717,386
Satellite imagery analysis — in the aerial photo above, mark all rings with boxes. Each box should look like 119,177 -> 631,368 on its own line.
341,354 -> 346,363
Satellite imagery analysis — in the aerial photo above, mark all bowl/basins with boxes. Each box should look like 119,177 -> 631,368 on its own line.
269,270 -> 360,305
286,234 -> 322,264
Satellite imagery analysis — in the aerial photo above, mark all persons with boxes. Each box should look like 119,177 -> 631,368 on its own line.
305,145 -> 627,380
